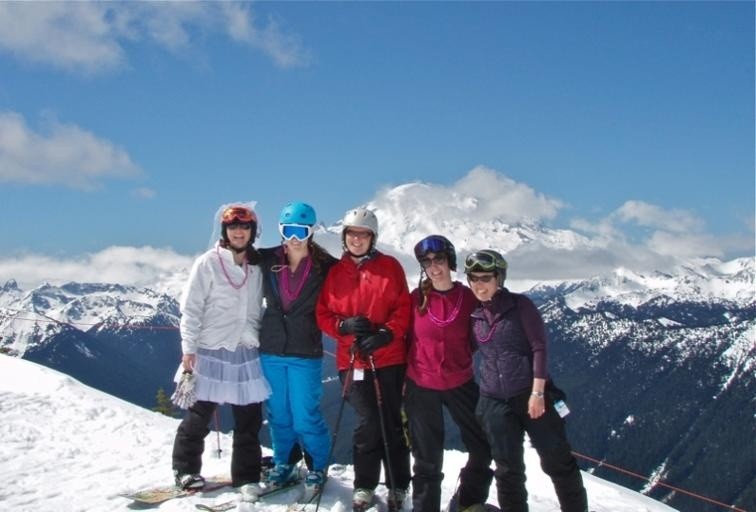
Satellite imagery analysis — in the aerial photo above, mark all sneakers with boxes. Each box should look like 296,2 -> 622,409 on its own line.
237,482 -> 263,499
351,484 -> 374,508
384,483 -> 411,512
259,462 -> 301,489
173,468 -> 206,490
299,468 -> 327,491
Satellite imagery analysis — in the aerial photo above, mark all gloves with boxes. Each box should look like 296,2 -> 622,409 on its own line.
338,315 -> 372,336
169,367 -> 199,410
353,326 -> 395,358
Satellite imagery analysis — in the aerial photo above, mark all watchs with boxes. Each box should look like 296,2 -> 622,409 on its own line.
531,392 -> 544,399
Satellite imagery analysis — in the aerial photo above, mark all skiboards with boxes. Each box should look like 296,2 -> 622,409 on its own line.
195,463 -> 346,512
353,486 -> 404,511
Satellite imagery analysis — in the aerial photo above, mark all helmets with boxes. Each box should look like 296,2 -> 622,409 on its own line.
219,204 -> 258,248
414,234 -> 457,273
463,249 -> 508,290
277,200 -> 319,228
339,206 -> 379,257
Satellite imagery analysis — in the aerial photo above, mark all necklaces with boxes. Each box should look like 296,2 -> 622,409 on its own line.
425,281 -> 464,327
217,249 -> 248,289
476,319 -> 497,344
282,255 -> 313,301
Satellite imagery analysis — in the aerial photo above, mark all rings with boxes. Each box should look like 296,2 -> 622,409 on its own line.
542,409 -> 546,413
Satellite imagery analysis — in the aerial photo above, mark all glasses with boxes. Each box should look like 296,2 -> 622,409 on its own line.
467,273 -> 498,284
420,255 -> 449,269
342,226 -> 371,239
279,223 -> 317,242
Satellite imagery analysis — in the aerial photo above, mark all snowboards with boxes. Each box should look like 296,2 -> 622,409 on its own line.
118,476 -> 230,504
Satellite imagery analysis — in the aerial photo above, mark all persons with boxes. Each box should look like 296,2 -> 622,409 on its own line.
246,202 -> 342,490
464,249 -> 588,512
171,206 -> 274,495
401,236 -> 492,512
315,207 -> 412,508
